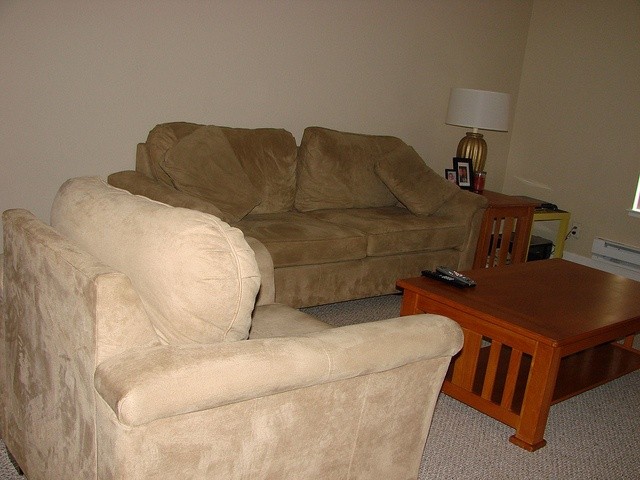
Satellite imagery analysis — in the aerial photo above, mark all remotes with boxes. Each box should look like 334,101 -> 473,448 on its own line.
436,265 -> 477,288
422,269 -> 457,284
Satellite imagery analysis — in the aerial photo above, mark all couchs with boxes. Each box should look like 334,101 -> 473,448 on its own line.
108,121 -> 489,309
0,180 -> 465,480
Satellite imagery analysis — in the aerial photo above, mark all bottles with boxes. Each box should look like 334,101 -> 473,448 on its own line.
473,170 -> 487,193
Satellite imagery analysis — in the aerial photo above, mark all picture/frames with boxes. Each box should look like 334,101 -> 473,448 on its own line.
446,169 -> 458,185
453,157 -> 472,189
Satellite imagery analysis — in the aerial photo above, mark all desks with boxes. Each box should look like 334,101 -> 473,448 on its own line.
474,190 -> 534,270
513,196 -> 572,258
397,258 -> 640,452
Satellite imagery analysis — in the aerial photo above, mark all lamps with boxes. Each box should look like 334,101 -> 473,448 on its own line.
447,87 -> 511,172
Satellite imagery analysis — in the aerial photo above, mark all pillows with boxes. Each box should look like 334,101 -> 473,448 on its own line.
374,146 -> 459,217
294,126 -> 404,212
162,126 -> 260,222
143,122 -> 295,212
53,180 -> 259,347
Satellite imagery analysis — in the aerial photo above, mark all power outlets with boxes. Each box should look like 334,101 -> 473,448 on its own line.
571,224 -> 581,238
